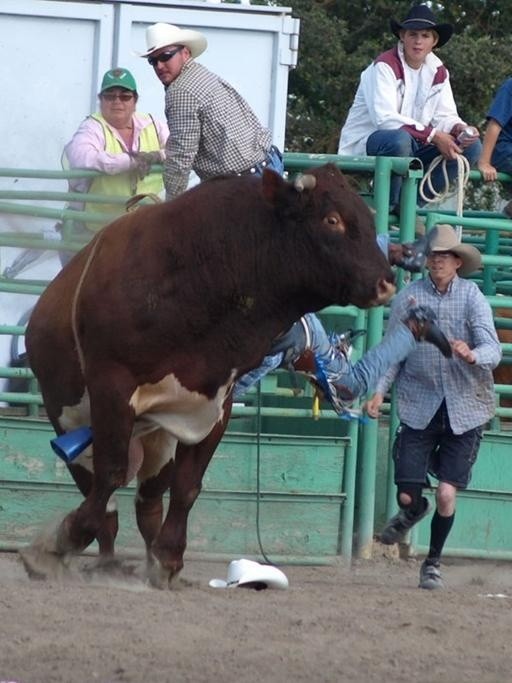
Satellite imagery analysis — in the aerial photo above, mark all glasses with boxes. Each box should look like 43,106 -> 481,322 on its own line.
103,95 -> 133,101
148,50 -> 180,65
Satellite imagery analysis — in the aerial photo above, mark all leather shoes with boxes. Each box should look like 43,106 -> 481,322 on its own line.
400,236 -> 428,272
410,305 -> 454,358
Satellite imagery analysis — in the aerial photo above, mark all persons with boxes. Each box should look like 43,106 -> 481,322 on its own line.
366,222 -> 501,591
58,67 -> 171,269
138,23 -> 285,202
231,302 -> 453,415
477,76 -> 512,218
337,4 -> 479,239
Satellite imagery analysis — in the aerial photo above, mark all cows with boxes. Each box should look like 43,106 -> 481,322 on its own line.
19,161 -> 397,591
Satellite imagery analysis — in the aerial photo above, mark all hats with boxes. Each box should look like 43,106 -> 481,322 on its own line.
210,559 -> 290,590
138,23 -> 208,58
100,68 -> 137,93
427,225 -> 482,276
391,6 -> 455,48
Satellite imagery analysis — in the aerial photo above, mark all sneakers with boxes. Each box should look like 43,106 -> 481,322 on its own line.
380,498 -> 432,545
420,558 -> 443,589
